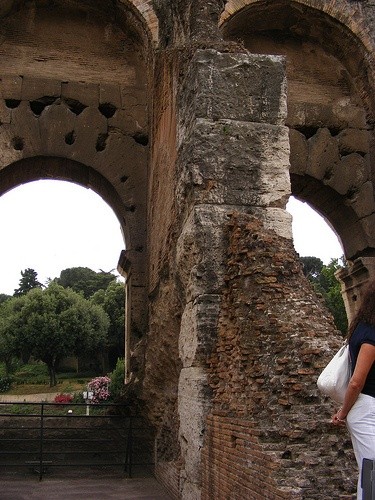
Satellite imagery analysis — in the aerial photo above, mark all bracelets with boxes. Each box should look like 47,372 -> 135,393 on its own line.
336,408 -> 346,423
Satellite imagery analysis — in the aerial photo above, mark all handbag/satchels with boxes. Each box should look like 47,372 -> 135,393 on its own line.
317,343 -> 350,403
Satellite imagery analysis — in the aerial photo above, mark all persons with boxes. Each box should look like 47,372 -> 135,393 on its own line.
331,281 -> 374,500
